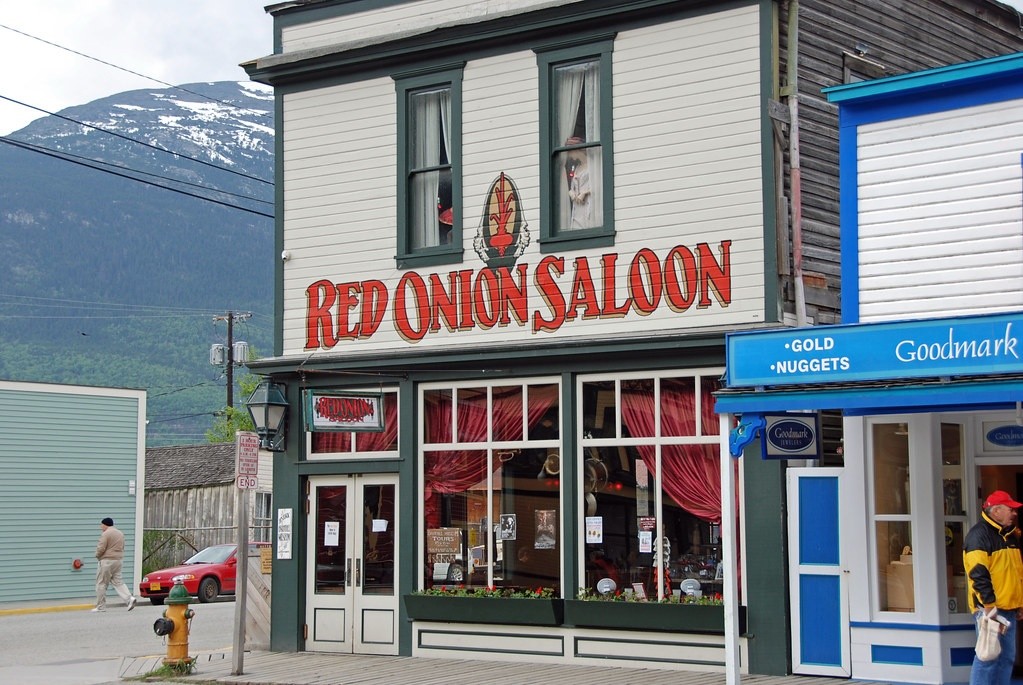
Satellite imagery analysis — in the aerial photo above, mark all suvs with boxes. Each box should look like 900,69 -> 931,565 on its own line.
470,539 -> 503,581
432,544 -> 474,585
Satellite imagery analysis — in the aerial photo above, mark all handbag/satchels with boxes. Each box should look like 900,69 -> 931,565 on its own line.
975,607 -> 1002,662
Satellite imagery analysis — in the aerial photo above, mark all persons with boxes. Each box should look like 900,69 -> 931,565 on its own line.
963,491 -> 1023,685
512,545 -> 539,591
565,137 -> 592,229
91,517 -> 137,612
585,548 -> 621,598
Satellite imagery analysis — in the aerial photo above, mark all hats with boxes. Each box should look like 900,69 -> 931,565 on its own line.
982,491 -> 1023,510
101,518 -> 113,526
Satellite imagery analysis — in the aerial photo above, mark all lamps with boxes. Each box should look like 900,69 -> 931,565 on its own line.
246,377 -> 290,452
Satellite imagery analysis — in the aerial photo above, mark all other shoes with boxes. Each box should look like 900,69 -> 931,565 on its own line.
92,607 -> 106,612
127,597 -> 136,611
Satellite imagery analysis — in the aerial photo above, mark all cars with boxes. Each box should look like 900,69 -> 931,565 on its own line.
139,543 -> 271,604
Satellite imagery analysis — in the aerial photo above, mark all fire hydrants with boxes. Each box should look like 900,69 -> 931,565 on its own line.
154,578 -> 195,671
